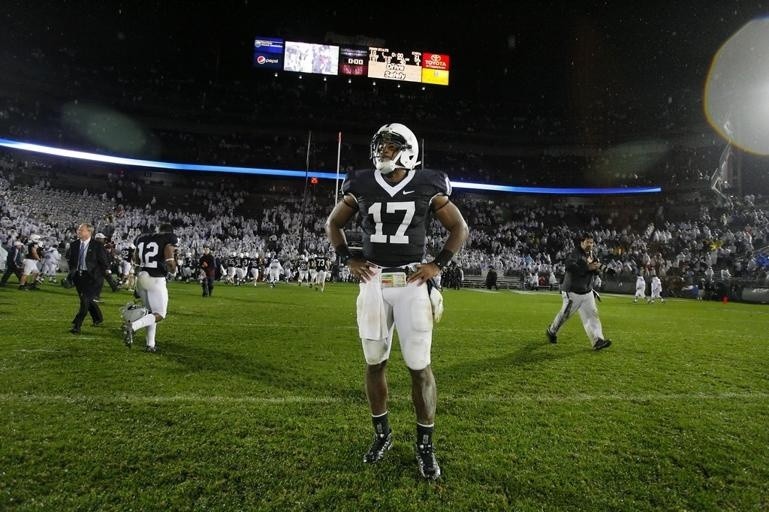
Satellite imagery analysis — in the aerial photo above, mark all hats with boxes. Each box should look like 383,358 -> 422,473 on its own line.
95,233 -> 106,239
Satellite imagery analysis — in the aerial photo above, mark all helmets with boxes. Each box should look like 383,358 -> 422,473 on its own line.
230,251 -> 271,260
369,123 -> 420,176
300,253 -> 317,261
123,303 -> 147,323
29,234 -> 44,249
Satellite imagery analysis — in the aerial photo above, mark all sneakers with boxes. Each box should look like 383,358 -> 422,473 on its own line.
416,440 -> 441,481
364,430 -> 394,464
594,339 -> 611,351
121,323 -> 133,349
546,326 -> 558,344
143,345 -> 162,353
18,283 -> 39,290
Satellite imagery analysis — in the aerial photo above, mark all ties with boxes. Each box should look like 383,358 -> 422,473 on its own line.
80,243 -> 84,266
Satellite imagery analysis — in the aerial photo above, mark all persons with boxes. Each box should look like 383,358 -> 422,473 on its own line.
122,225 -> 177,352
61,224 -> 109,335
547,231 -> 611,349
323,123 -> 469,481
2,44 -> 768,303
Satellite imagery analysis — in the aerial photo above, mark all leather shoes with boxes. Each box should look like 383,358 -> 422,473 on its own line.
91,321 -> 103,326
69,326 -> 80,334
